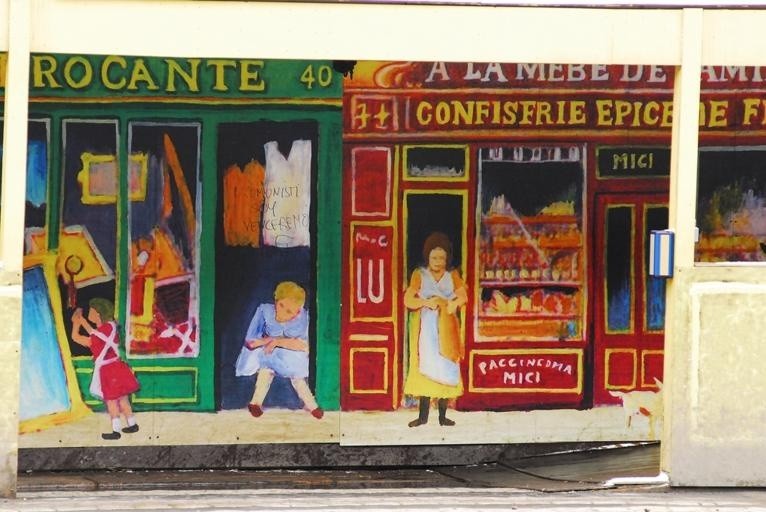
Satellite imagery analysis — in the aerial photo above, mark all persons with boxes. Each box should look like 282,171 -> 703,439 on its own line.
71,298 -> 141,440
235,281 -> 324,419
402,232 -> 468,426
